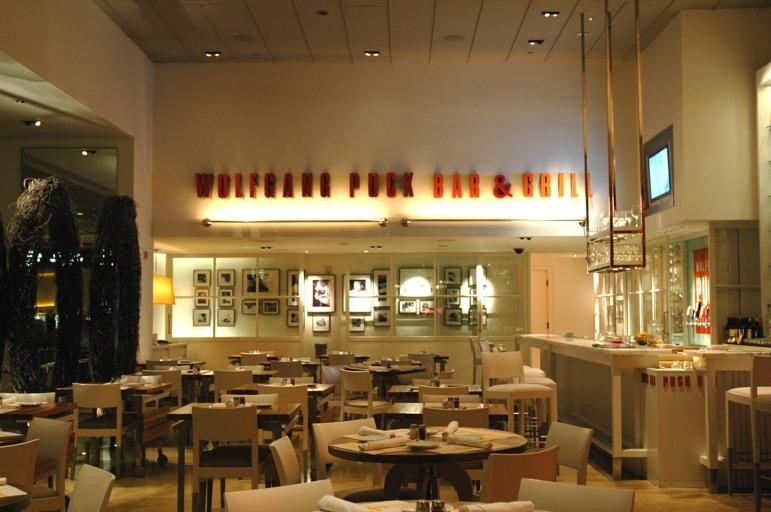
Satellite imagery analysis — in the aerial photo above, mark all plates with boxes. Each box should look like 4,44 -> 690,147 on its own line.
407,442 -> 438,450
18,401 -> 43,407
250,402 -> 272,408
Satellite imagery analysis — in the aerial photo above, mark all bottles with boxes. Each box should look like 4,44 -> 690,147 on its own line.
686,300 -> 710,347
283,376 -> 296,386
447,397 -> 453,409
726,312 -> 763,345
453,397 -> 459,409
234,396 -> 246,408
409,423 -> 418,441
416,500 -> 448,512
418,425 -> 427,440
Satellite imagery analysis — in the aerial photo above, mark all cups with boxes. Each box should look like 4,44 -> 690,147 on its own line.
623,336 -> 633,347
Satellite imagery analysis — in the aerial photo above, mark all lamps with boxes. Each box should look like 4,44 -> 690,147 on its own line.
153,275 -> 175,306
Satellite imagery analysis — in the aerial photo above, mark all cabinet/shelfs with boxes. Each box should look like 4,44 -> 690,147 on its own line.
586,1 -> 646,274
644,366 -> 730,492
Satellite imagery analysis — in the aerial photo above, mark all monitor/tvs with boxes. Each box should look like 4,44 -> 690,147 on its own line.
647,144 -> 670,201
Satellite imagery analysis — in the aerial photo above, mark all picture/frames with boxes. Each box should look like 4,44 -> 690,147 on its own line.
193,263 -> 487,332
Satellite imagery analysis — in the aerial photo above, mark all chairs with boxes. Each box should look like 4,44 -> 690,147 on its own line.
724,352 -> 771,512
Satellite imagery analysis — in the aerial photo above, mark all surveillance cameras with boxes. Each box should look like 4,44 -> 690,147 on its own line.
513,248 -> 525,255
